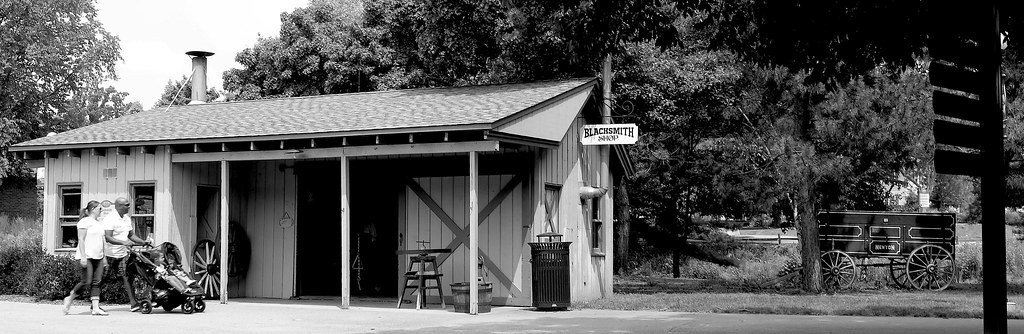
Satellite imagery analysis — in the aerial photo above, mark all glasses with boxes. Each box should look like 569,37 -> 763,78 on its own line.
94,207 -> 102,211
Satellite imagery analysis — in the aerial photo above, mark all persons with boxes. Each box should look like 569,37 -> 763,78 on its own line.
149,251 -> 197,295
63,201 -> 109,316
90,197 -> 152,312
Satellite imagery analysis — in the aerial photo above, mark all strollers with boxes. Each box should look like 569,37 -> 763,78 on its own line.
126,241 -> 206,315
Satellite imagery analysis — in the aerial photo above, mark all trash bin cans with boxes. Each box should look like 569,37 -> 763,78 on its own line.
527,232 -> 574,311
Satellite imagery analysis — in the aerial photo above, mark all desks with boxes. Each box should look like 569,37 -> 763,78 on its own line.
397,256 -> 446,310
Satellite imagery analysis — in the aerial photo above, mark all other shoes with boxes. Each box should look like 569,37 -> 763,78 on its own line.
90,305 -> 105,312
64,297 -> 70,315
92,308 -> 108,315
131,302 -> 141,311
